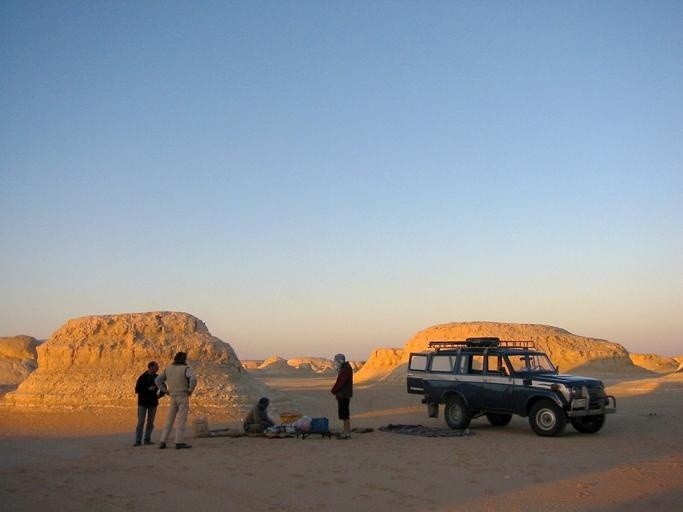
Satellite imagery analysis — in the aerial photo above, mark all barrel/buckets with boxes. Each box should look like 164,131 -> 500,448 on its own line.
192,415 -> 210,436
280,411 -> 302,424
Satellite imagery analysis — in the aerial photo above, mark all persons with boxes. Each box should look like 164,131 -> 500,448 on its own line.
153,351 -> 198,450
242,395 -> 275,433
327,353 -> 355,439
133,360 -> 166,448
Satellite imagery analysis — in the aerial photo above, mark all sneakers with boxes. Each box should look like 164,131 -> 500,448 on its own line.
338,435 -> 351,439
176,444 -> 191,449
134,442 -> 140,446
159,443 -> 166,449
144,441 -> 153,444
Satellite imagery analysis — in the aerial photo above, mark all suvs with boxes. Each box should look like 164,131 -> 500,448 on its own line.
406,336 -> 617,438
